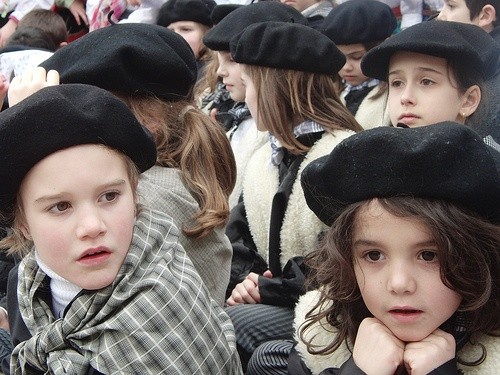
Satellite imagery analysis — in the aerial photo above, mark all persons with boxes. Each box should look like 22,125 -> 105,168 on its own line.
0,0 -> 500,375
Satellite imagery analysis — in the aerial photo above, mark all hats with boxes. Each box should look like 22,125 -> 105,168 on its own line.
321,0 -> 395,44
230,20 -> 346,77
209,4 -> 242,23
361,19 -> 500,78
0,84 -> 157,210
203,1 -> 308,51
156,0 -> 216,28
40,23 -> 197,103
301,121 -> 500,226
